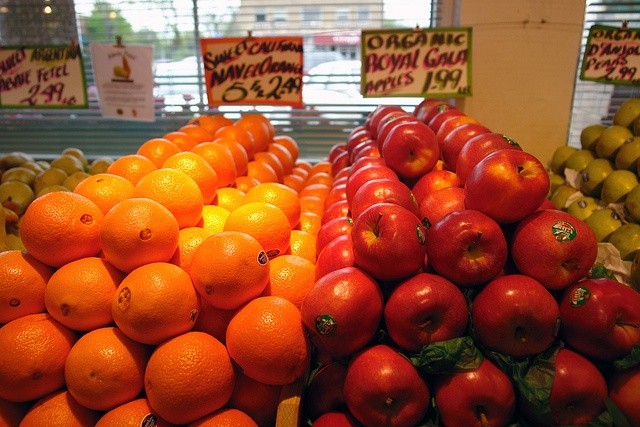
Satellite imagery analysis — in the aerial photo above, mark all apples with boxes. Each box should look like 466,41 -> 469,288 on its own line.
301,99 -> 640,427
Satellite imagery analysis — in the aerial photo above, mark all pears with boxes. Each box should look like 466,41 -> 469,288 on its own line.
0,148 -> 115,253
546,99 -> 640,295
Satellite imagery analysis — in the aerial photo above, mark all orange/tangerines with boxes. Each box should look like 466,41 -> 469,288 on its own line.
0,114 -> 334,427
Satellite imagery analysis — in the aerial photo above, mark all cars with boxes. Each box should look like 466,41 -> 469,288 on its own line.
288,57 -> 426,132
150,56 -> 283,122
300,49 -> 343,77
